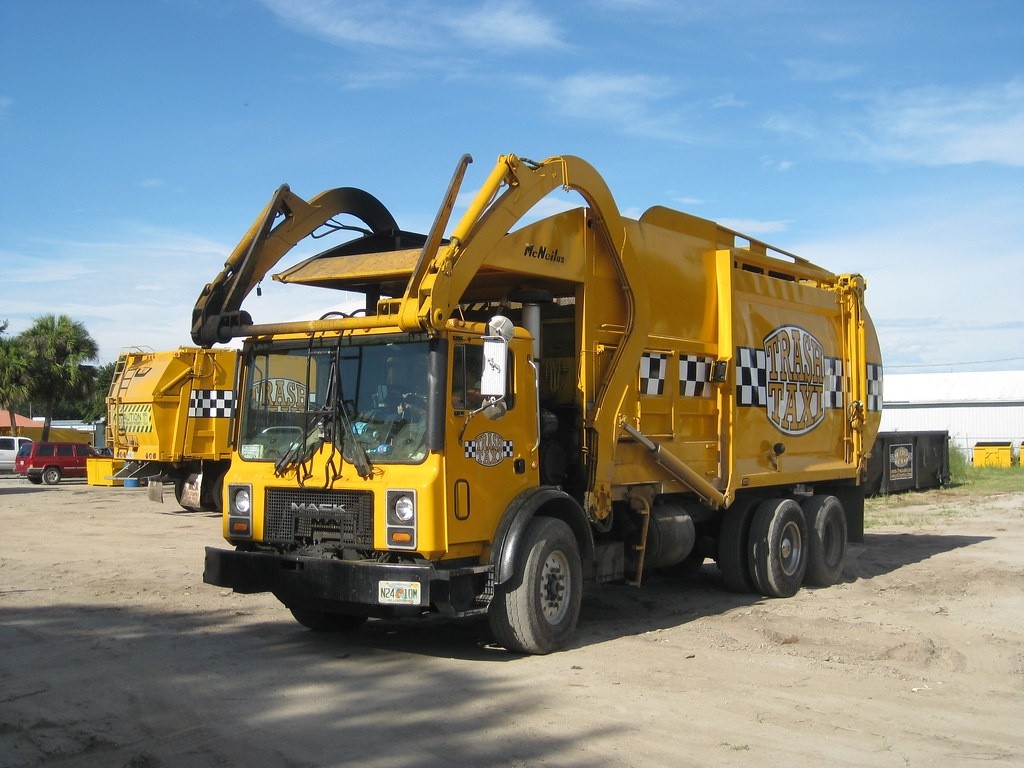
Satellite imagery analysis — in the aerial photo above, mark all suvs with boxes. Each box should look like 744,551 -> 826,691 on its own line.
13,441 -> 114,485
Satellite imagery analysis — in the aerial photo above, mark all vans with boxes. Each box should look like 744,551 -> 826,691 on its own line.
0,436 -> 40,471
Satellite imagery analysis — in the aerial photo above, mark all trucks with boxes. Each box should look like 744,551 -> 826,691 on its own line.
105,345 -> 317,513
190,155 -> 885,656
